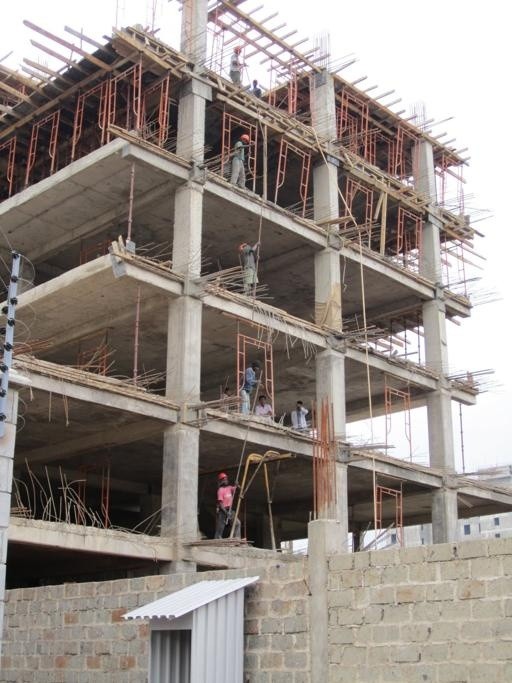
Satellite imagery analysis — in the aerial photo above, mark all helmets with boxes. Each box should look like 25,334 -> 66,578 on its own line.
217,472 -> 228,480
241,134 -> 249,142
251,360 -> 262,369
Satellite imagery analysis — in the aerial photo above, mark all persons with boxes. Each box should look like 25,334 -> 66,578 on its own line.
253,80 -> 261,98
215,473 -> 241,539
221,360 -> 273,417
230,134 -> 252,189
230,46 -> 248,87
239,241 -> 261,300
291,400 -> 308,429
